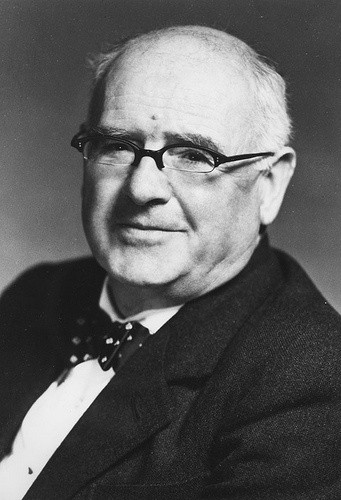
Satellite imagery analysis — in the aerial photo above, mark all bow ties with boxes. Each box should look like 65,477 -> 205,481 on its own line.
59,304 -> 153,373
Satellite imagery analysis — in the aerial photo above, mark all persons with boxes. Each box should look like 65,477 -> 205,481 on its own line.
0,24 -> 340,499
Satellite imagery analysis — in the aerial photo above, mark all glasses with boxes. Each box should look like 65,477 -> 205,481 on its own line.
69,126 -> 274,173
